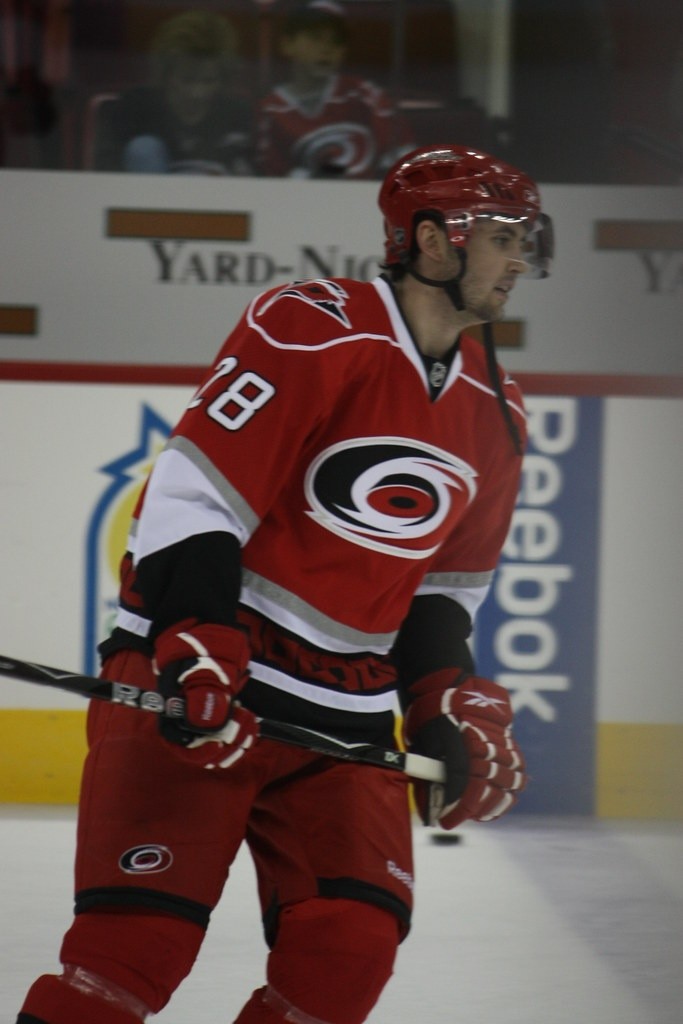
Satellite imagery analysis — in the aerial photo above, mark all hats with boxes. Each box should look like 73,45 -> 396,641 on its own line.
279,1 -> 356,50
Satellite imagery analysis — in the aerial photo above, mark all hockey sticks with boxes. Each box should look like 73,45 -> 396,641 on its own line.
0,650 -> 447,785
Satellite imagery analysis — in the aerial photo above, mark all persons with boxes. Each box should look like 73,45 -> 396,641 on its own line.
0,1 -> 419,179
14,149 -> 539,1024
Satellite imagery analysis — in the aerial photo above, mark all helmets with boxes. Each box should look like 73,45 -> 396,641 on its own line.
378,143 -> 554,277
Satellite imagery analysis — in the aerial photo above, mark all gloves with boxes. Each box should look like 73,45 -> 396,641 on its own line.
399,672 -> 527,829
152,623 -> 261,772
